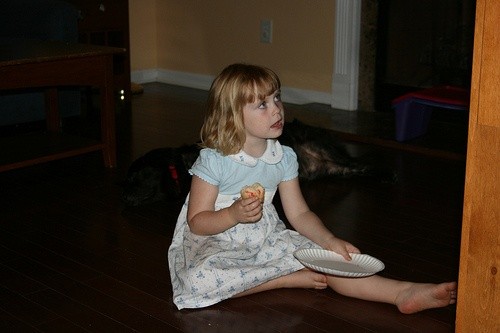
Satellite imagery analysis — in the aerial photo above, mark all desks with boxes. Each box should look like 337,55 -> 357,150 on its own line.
0,39 -> 126,183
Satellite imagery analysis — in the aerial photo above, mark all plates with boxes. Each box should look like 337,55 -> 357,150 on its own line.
293,248 -> 385,278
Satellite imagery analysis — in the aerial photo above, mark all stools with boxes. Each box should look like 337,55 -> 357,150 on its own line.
392,84 -> 470,149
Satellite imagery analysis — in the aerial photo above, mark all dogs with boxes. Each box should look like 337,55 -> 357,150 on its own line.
122,118 -> 400,212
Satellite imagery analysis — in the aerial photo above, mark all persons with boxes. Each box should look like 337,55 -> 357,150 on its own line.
167,63 -> 456,314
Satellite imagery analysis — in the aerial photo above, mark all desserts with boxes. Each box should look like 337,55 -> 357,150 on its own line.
240,183 -> 264,209
